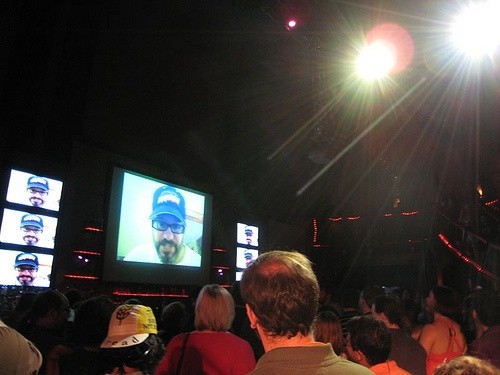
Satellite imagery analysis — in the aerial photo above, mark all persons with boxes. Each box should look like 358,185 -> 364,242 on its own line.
5,252 -> 49,288
0,320 -> 45,375
0,262 -> 498,365
99,305 -> 167,374
160,285 -> 255,375
344,316 -> 411,374
433,358 -> 499,374
241,226 -> 258,246
10,214 -> 51,248
14,176 -> 59,212
47,297 -> 115,375
238,249 -> 255,268
239,251 -> 375,375
123,186 -> 201,266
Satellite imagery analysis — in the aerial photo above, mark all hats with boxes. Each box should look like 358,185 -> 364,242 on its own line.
97,304 -> 158,348
245,226 -> 253,234
20,214 -> 43,229
149,187 -> 185,223
27,176 -> 49,192
244,250 -> 253,258
14,253 -> 38,270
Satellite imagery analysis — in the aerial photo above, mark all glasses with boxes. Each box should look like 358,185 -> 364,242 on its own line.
246,257 -> 252,260
23,227 -> 41,232
246,233 -> 252,236
17,266 -> 38,272
29,188 -> 47,196
152,220 -> 184,235
